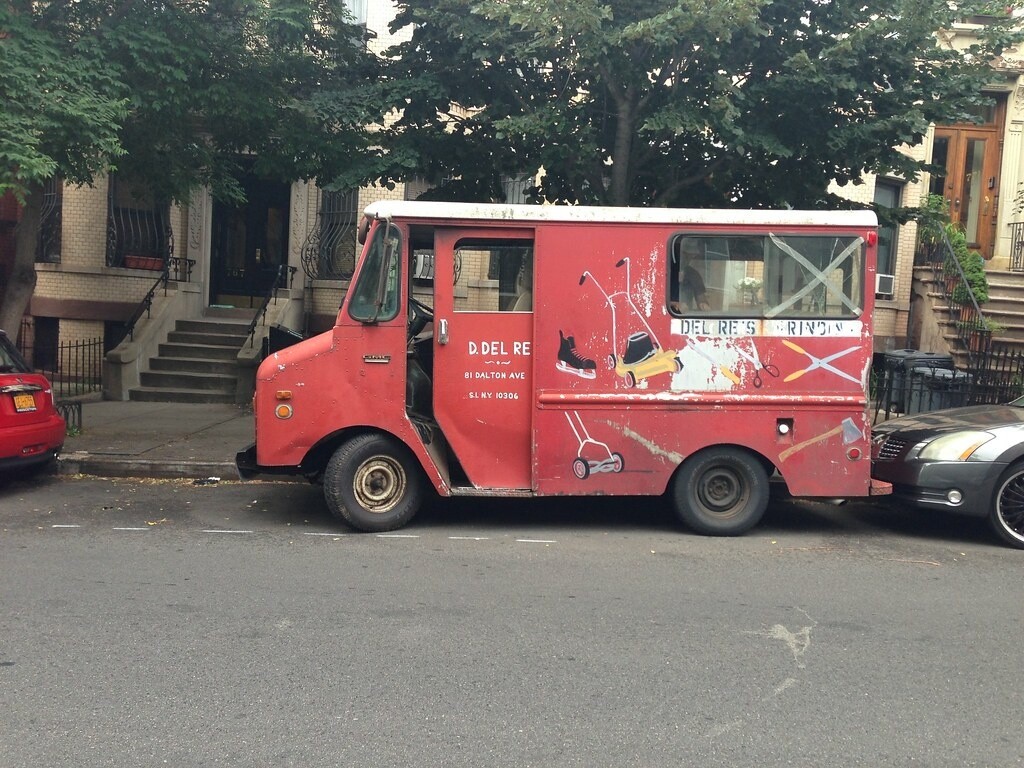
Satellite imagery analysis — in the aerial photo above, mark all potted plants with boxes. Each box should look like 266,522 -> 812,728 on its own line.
960,314 -> 1009,352
943,246 -> 968,294
952,283 -> 988,322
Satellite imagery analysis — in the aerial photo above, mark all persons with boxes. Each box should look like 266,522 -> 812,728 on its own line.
667,236 -> 712,316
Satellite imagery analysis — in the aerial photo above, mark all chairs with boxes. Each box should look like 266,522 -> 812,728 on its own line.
507,268 -> 533,311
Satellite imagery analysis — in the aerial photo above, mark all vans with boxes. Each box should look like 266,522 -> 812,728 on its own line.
235,200 -> 893,536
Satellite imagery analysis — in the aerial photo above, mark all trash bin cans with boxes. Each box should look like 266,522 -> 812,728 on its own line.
909,367 -> 973,414
881,348 -> 954,413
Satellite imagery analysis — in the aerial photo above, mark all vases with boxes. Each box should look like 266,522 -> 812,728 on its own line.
125,256 -> 163,270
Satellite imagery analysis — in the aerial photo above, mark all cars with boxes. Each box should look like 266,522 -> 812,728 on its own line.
867,395 -> 1024,550
0,329 -> 67,474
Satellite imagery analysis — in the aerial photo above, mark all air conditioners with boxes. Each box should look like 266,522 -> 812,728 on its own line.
411,255 -> 435,278
875,273 -> 895,295
